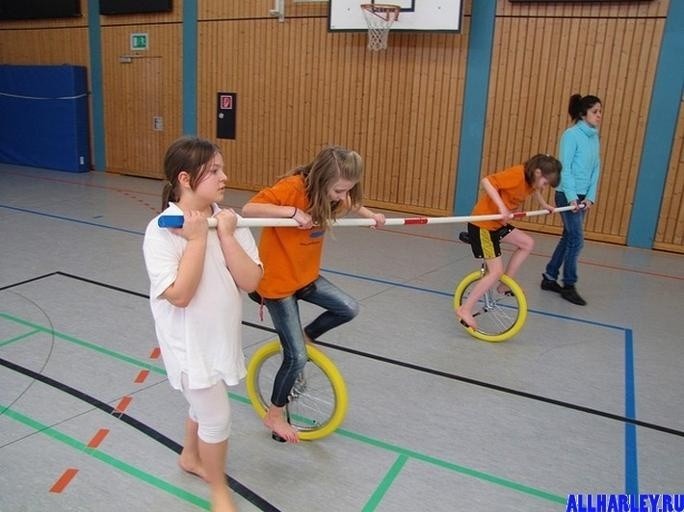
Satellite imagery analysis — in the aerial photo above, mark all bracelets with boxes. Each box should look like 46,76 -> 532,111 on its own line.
287,206 -> 298,226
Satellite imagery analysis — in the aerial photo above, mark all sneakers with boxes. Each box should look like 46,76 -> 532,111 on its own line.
559,284 -> 587,307
538,273 -> 562,293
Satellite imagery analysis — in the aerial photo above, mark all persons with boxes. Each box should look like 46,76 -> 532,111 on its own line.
242,146 -> 387,444
142,137 -> 264,512
455,154 -> 561,330
541,93 -> 603,305
